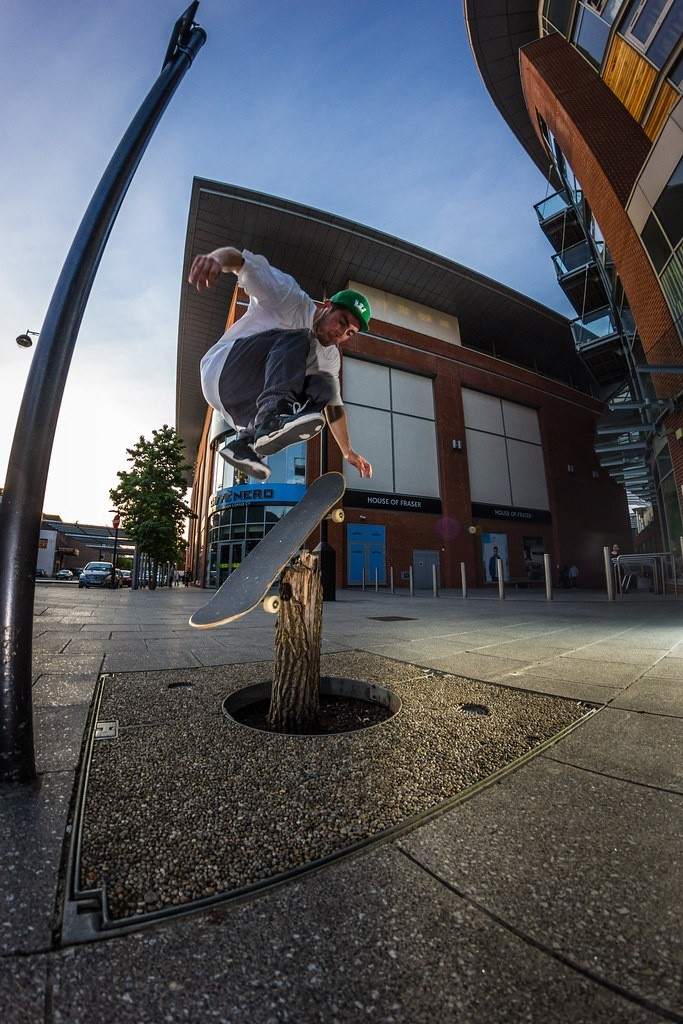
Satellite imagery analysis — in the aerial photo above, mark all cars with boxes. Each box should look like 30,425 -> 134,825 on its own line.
121,570 -> 133,588
139,571 -> 167,587
115,568 -> 123,588
78,562 -> 113,588
55,570 -> 73,581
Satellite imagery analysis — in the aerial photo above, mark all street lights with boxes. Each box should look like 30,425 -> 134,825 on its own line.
109,510 -> 121,585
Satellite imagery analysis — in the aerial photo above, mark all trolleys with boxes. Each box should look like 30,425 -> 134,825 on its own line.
614,558 -> 658,593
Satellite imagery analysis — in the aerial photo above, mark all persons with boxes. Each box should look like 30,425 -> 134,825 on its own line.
553,562 -> 580,590
187,247 -> 373,482
610,543 -> 623,592
488,546 -> 503,582
168,567 -> 192,588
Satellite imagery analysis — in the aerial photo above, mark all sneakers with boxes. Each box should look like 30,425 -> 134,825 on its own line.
219,440 -> 271,482
255,400 -> 325,456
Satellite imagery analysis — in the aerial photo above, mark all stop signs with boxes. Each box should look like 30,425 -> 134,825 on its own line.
113,515 -> 120,528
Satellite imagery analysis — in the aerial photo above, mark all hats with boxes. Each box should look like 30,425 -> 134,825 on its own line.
328,289 -> 370,331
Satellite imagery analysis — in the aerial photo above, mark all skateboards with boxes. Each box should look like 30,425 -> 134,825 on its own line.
189,473 -> 351,629
